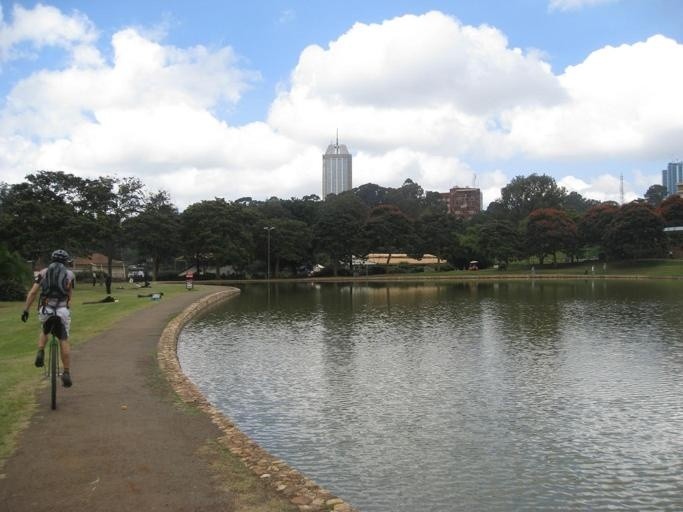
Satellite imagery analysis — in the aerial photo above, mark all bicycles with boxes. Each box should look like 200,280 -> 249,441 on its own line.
35,315 -> 70,410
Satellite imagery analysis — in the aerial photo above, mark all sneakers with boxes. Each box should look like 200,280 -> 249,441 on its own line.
61,372 -> 71,387
36,350 -> 45,367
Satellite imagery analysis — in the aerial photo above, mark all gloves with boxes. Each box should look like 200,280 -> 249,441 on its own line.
22,310 -> 29,322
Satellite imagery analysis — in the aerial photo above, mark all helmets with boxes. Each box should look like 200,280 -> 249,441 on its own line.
51,250 -> 69,265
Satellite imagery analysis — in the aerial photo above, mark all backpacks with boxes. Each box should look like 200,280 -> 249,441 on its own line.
42,263 -> 69,301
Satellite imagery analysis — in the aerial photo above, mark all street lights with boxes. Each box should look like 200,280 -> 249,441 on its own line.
263,226 -> 276,280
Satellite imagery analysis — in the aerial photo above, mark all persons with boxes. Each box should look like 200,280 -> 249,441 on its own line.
92,271 -> 97,287
21,250 -> 74,387
